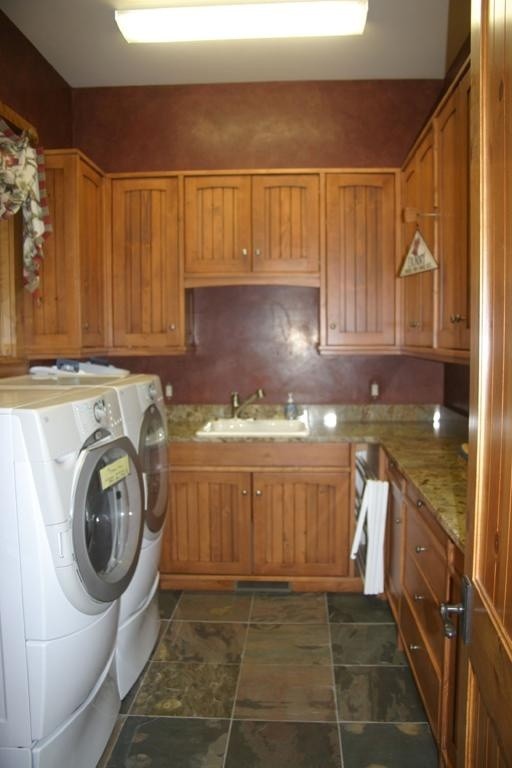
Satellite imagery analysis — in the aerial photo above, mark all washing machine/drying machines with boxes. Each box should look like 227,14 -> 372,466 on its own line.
1,372 -> 173,702
0,385 -> 150,768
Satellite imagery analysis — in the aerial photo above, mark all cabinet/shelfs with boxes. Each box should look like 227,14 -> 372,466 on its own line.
28,149 -> 105,362
398,478 -> 454,746
382,450 -> 404,619
184,171 -> 323,288
399,118 -> 435,360
322,168 -> 399,354
106,172 -> 182,357
156,440 -> 356,593
435,57 -> 472,363
1,211 -> 28,374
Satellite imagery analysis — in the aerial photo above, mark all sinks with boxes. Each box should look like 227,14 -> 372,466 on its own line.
195,417 -> 311,437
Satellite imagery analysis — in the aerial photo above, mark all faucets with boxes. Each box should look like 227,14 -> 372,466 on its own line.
229,388 -> 266,418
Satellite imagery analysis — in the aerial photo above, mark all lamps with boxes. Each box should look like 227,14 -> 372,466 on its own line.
113,0 -> 369,45
